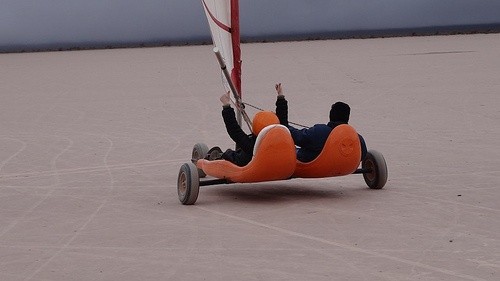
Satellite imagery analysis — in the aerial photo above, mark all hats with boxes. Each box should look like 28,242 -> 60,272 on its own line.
329,101 -> 351,123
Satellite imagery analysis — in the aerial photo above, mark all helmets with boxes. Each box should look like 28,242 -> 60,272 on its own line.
252,110 -> 280,135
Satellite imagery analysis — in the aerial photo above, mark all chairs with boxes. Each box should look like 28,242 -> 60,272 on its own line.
197,124 -> 297,183
294,124 -> 362,178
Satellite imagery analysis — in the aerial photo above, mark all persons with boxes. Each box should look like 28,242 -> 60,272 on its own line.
275,82 -> 368,164
209,90 -> 281,167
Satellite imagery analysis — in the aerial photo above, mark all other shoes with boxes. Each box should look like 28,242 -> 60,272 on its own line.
210,151 -> 219,161
